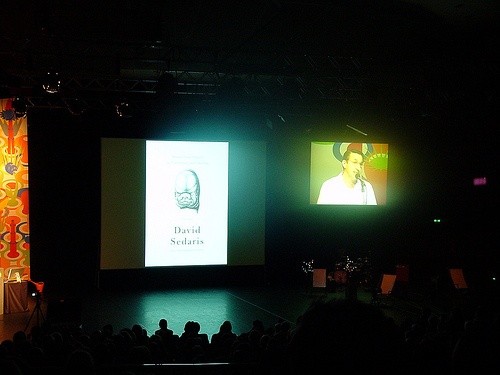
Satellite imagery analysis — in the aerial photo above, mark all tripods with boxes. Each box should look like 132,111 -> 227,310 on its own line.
22,294 -> 45,331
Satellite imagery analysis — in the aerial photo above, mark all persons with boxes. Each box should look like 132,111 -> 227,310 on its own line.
316,149 -> 379,206
0,303 -> 500,375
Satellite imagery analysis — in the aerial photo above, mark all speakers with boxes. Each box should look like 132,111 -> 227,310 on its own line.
45,298 -> 83,328
